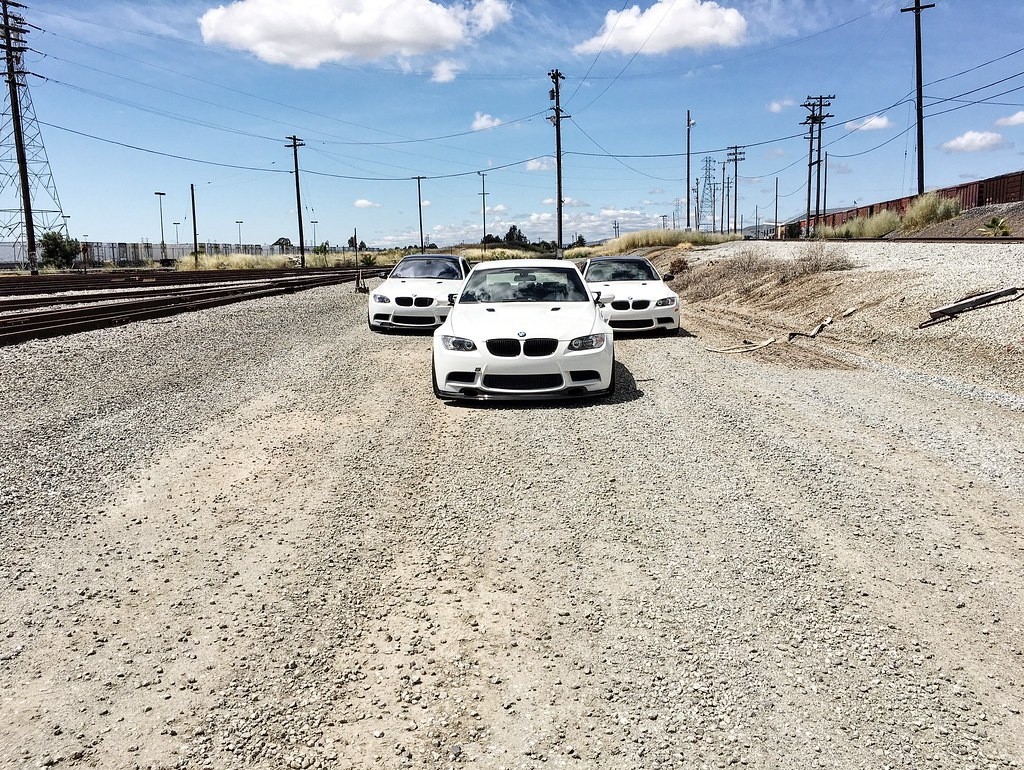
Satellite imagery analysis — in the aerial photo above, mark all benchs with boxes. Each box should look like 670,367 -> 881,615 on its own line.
486,282 -> 570,301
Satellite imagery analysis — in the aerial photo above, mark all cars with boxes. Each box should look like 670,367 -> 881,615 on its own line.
578,256 -> 683,335
431,257 -> 616,403
368,254 -> 473,336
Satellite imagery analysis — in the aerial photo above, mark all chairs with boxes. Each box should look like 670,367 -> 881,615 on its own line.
591,270 -> 604,282
488,284 -> 509,299
545,284 -> 567,300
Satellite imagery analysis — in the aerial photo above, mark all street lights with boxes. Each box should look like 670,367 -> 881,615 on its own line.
154,192 -> 166,244
63,216 -> 71,240
84,235 -> 88,245
236,221 -> 244,242
311,220 -> 318,256
173,222 -> 181,244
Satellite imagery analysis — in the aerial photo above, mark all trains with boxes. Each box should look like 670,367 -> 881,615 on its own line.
797,170 -> 1024,238
